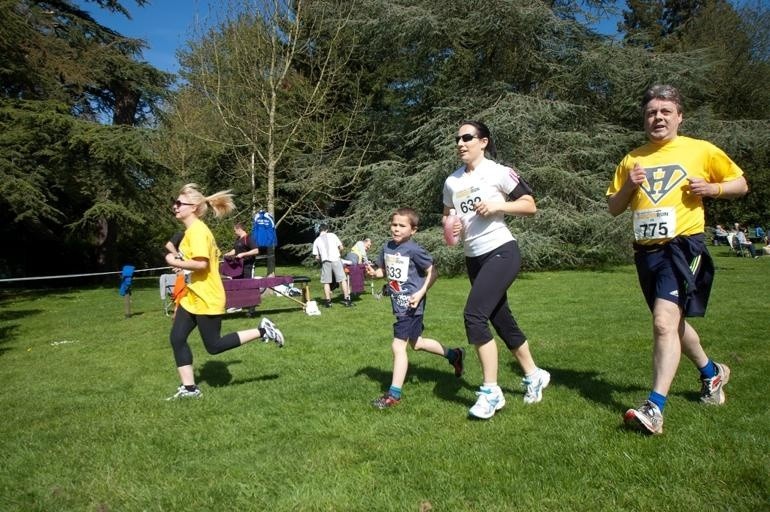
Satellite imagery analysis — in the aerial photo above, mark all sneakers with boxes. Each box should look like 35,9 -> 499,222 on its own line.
323,298 -> 333,308
520,367 -> 552,405
162,386 -> 203,403
369,394 -> 403,410
448,346 -> 468,379
621,397 -> 664,438
342,296 -> 357,309
258,316 -> 285,348
697,360 -> 731,406
468,384 -> 509,421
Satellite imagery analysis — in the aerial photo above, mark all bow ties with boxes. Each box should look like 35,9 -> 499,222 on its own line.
493,411 -> 494,413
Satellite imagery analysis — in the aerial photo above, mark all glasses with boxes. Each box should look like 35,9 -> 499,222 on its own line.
455,133 -> 477,147
174,199 -> 195,209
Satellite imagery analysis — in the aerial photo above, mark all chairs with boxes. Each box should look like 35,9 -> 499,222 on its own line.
732,234 -> 749,258
726,234 -> 736,257
159,273 -> 179,318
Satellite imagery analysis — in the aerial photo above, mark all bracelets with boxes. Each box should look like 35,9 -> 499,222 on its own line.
712,182 -> 722,199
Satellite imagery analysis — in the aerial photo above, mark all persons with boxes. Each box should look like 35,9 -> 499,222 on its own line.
341,237 -> 372,268
160,183 -> 284,399
361,208 -> 465,413
313,224 -> 350,308
442,122 -> 551,422
605,86 -> 751,438
714,222 -> 770,259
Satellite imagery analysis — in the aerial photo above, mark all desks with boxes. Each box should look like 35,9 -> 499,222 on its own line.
331,263 -> 375,301
217,274 -> 311,321
